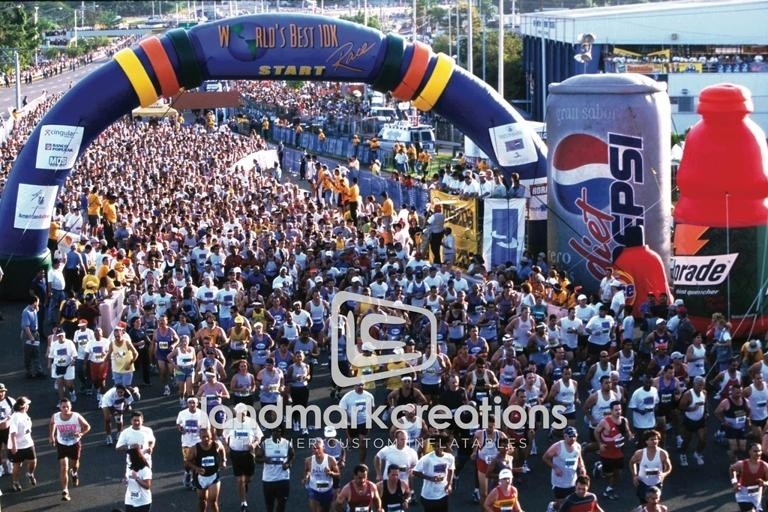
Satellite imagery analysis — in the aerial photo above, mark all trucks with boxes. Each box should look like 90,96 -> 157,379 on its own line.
375,119 -> 437,156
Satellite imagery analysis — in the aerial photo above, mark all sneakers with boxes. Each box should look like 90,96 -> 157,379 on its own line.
0,379 -> 308,508
452,424 -> 725,512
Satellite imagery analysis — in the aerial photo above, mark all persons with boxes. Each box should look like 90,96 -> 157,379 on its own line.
1,0 -> 767,512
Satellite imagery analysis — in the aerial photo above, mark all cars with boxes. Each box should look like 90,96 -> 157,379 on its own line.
144,14 -> 169,24
362,23 -> 436,133
189,8 -> 224,21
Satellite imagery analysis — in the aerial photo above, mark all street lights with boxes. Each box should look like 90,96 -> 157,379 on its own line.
0,5 -> 22,13
23,7 -> 63,28
574,33 -> 596,74
70,4 -> 99,47
